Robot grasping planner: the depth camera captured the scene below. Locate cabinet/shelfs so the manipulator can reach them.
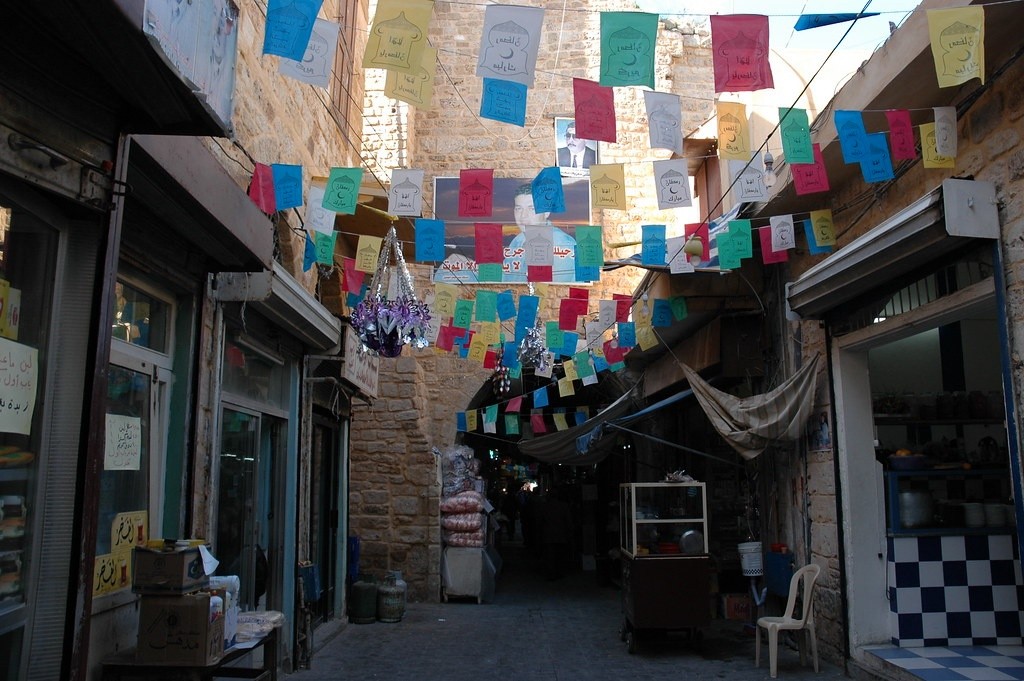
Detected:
[615,481,709,562]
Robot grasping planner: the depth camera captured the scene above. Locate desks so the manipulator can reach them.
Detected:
[98,627,277,681]
[443,544,490,604]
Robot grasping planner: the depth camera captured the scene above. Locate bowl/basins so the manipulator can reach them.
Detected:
[771,544,786,552]
[889,455,924,471]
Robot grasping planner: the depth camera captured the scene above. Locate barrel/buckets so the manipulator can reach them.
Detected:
[737,541,764,576]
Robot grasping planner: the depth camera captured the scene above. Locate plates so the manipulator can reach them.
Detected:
[679,530,703,553]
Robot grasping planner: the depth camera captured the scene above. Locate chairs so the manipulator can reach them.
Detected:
[755,564,821,678]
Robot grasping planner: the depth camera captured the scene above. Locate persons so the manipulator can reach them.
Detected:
[537,488,575,580]
[520,506,529,544]
[558,122,595,168]
[502,183,585,283]
[500,497,516,540]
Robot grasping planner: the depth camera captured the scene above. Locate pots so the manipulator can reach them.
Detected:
[899,491,932,527]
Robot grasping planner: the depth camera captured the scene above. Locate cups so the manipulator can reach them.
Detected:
[781,547,787,553]
[1007,506,1016,523]
[965,503,984,527]
[985,505,1005,527]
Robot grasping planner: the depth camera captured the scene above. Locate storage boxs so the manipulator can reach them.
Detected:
[134,575,237,666]
[130,541,211,596]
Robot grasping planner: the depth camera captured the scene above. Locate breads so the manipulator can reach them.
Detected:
[0,443,34,585]
